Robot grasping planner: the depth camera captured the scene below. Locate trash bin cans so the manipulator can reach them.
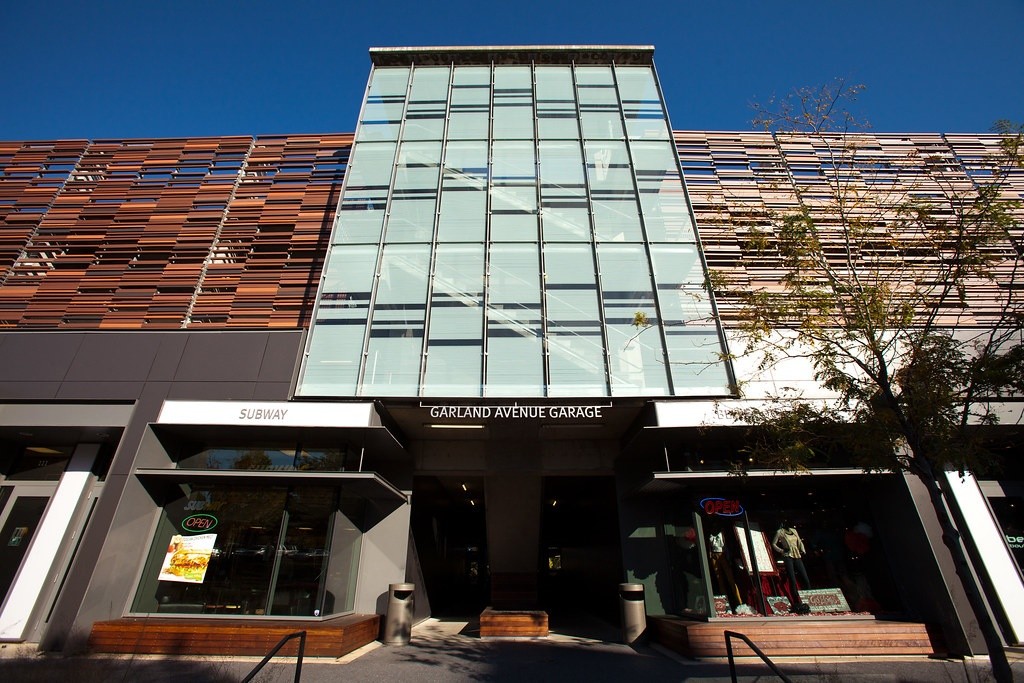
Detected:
[619,583,647,646]
[383,584,414,646]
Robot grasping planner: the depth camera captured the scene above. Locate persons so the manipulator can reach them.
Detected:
[709,521,742,606]
[772,517,812,613]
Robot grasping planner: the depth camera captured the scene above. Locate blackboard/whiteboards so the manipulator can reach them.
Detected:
[731,517,780,577]
